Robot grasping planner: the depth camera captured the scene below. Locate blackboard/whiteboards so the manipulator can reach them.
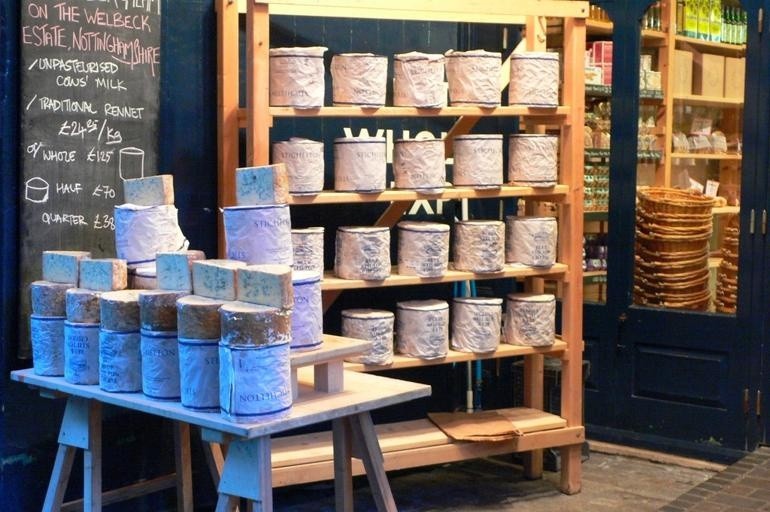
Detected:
[19,1,161,360]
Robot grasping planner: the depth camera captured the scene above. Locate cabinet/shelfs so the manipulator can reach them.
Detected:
[676,35,744,268]
[213,1,587,511]
[585,2,672,306]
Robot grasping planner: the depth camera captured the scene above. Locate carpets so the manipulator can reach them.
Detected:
[676,446,769,499]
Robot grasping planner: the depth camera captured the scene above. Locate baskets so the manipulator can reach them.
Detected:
[713,226,741,314]
[638,203,714,227]
[635,219,713,236]
[635,228,713,252]
[638,188,715,214]
[632,264,713,312]
[634,240,710,263]
[634,251,709,274]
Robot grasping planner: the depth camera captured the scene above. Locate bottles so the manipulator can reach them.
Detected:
[721,6,748,45]
[590,5,610,22]
[641,2,660,32]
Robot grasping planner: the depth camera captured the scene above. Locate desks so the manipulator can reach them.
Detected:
[286,334,371,400]
[11,367,433,511]
